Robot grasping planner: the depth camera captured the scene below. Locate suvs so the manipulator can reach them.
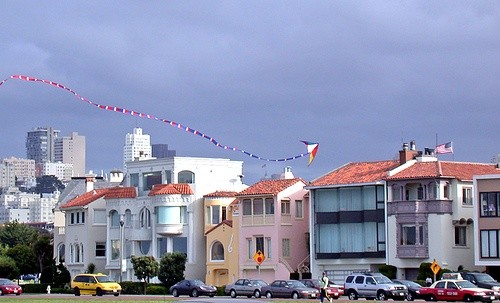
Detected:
[344,273,408,300]
[461,273,500,299]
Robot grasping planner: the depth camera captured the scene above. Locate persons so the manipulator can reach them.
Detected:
[43,285,58,298]
[320,271,332,303]
[426,274,432,286]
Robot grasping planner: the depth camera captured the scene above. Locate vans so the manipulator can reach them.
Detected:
[441,272,462,280]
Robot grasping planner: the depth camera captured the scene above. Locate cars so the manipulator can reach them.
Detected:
[20,274,36,281]
[70,274,122,296]
[169,279,216,297]
[0,278,22,295]
[391,279,423,301]
[297,278,342,299]
[327,281,345,295]
[262,280,320,299]
[420,280,491,302]
[225,279,268,298]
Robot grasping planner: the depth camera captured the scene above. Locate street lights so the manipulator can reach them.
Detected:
[120,221,124,272]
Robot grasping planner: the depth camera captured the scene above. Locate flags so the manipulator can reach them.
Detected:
[436,140,453,153]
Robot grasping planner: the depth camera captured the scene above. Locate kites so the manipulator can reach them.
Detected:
[298,139,319,166]
